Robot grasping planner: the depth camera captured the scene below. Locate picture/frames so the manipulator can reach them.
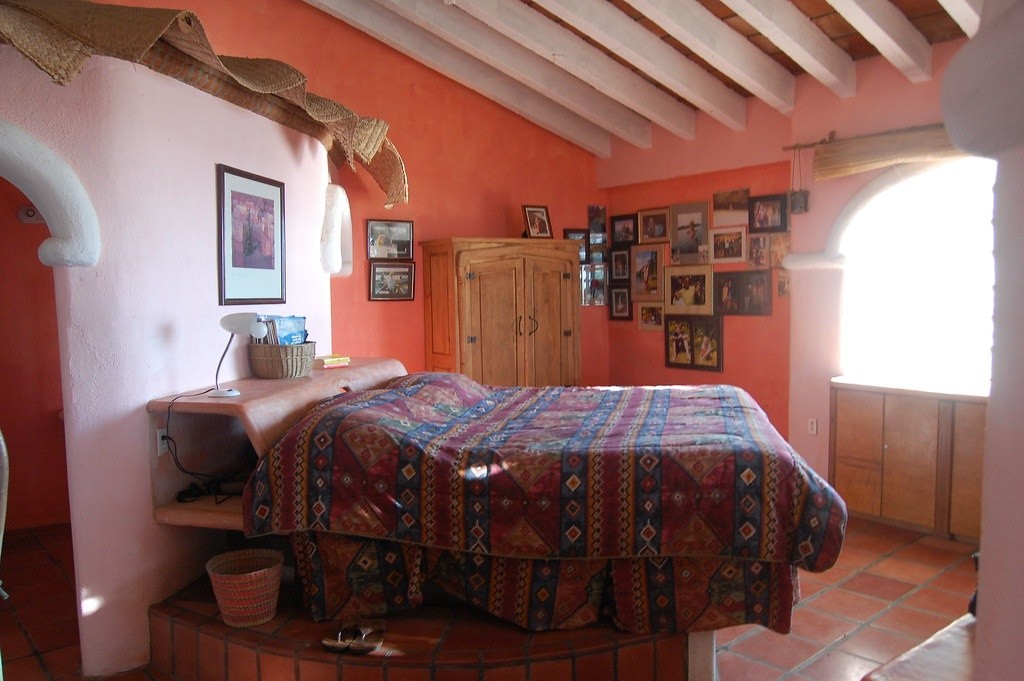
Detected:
[608,284,633,321]
[629,245,664,302]
[606,247,630,284]
[709,226,747,264]
[370,260,416,302]
[521,204,553,239]
[638,206,670,245]
[670,200,709,266]
[748,194,787,233]
[664,266,713,315]
[663,315,723,372]
[580,262,608,307]
[216,163,287,305]
[610,213,638,246]
[366,219,413,261]
[713,272,773,317]
[563,228,591,265]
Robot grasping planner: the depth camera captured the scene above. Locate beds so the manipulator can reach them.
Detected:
[146,356,847,636]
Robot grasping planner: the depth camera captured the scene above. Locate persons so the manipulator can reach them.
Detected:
[378,271,403,295]
[532,212,548,234]
[370,234,395,257]
[614,201,788,361]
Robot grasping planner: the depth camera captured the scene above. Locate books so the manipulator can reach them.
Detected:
[250,314,309,345]
[316,354,351,370]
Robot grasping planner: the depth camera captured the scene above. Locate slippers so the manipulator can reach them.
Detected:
[349,619,386,652]
[322,618,368,650]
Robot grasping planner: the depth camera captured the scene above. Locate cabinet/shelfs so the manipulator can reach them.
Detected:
[418,236,585,387]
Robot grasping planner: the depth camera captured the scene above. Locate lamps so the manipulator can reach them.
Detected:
[208,313,268,398]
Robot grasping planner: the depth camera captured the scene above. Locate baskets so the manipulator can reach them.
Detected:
[249,341,316,379]
[206,549,283,627]
